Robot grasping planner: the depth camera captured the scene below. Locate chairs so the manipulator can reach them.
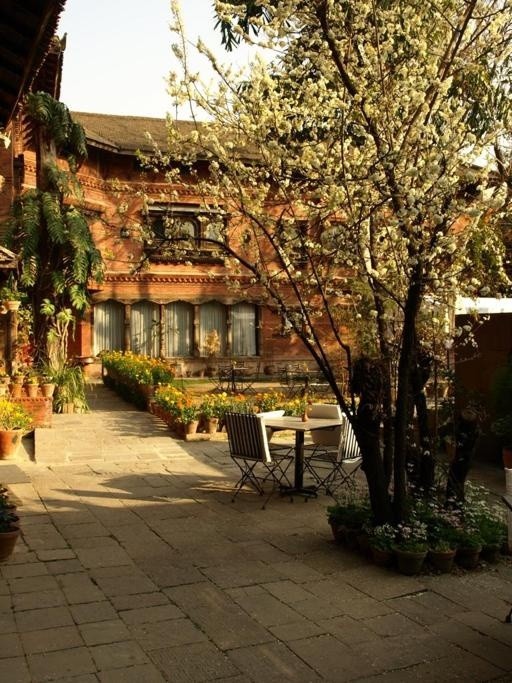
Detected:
[224,404,363,510]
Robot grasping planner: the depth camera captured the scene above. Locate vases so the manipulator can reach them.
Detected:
[376,547,482,573]
[151,404,219,439]
[328,521,352,544]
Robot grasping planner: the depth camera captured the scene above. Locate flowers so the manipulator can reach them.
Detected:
[327,477,506,549]
[147,386,303,419]
[100,349,176,384]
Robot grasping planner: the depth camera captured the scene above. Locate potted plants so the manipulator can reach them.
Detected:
[0,365,56,396]
[3,287,21,312]
[0,484,20,561]
[0,396,33,460]
